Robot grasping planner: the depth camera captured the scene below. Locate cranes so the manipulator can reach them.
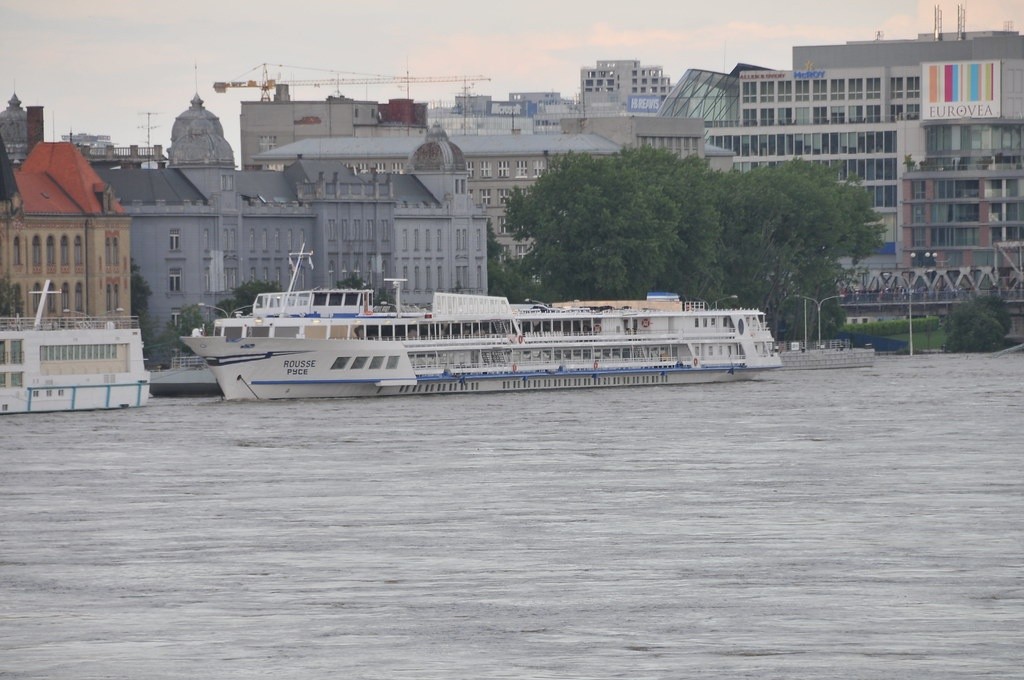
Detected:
[212,61,494,102]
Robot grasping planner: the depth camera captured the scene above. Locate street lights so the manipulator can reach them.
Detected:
[521,296,581,372]
[195,300,260,320]
[794,293,846,350]
[692,294,740,355]
[882,268,935,359]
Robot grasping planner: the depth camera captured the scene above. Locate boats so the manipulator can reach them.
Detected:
[180,237,784,404]
[1,279,152,417]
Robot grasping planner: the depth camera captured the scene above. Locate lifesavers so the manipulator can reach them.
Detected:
[594,325,602,333]
[642,318,650,328]
[593,362,598,369]
[518,334,523,344]
[512,364,517,372]
[694,358,697,365]
